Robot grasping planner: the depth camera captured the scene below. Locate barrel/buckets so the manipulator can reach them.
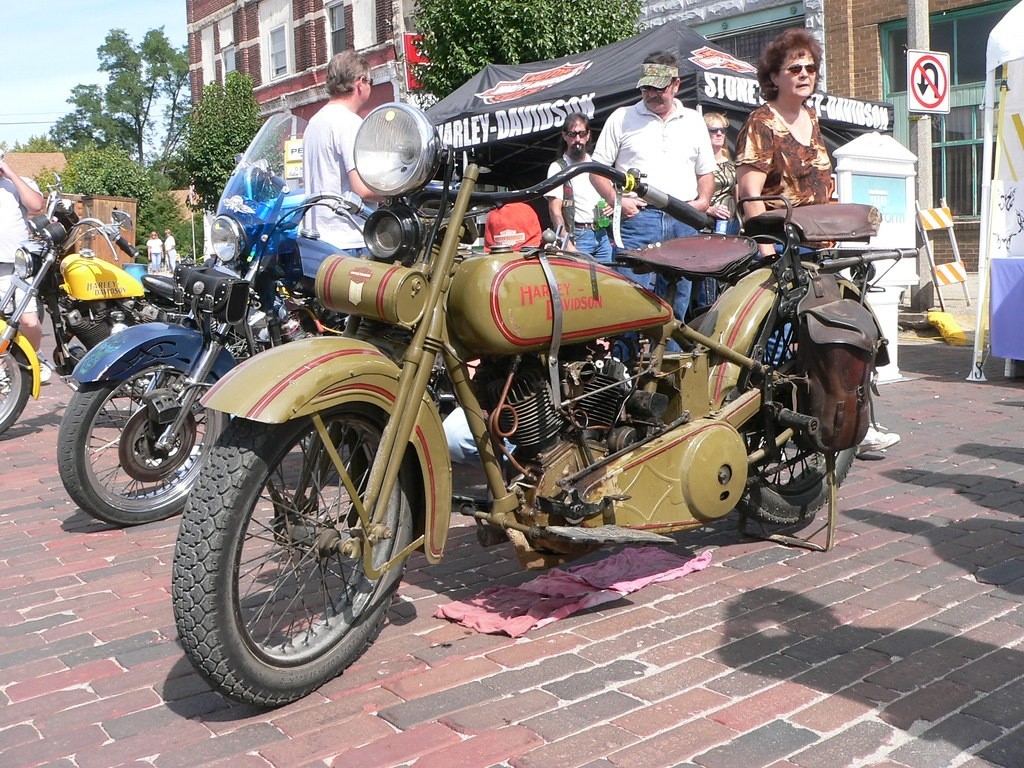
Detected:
[122,264,148,290]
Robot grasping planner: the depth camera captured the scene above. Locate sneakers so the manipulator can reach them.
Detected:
[853,423,900,457]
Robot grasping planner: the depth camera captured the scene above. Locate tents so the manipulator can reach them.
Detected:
[422,21,896,190]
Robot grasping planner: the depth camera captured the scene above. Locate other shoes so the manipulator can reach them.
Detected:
[0,369,8,386]
[37,351,54,383]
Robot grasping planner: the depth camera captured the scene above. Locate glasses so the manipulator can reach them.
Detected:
[358,77,374,88]
[708,127,730,134]
[562,130,588,139]
[641,86,668,93]
[779,64,818,75]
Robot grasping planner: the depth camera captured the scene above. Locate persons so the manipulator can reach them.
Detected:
[164,229,176,275]
[442,202,544,507]
[0,148,52,382]
[543,113,613,271]
[701,112,739,308]
[302,52,383,263]
[147,231,163,274]
[590,52,718,364]
[731,25,902,456]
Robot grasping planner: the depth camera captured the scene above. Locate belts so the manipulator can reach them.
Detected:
[637,204,652,209]
[575,223,596,230]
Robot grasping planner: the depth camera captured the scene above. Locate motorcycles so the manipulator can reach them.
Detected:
[1,101,922,710]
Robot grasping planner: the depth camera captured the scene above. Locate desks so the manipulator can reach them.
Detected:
[989,256,1024,378]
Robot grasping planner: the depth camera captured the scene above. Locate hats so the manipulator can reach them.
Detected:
[636,64,679,91]
[484,202,541,253]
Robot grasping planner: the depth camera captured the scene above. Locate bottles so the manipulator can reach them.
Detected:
[598,198,610,227]
[715,207,728,234]
